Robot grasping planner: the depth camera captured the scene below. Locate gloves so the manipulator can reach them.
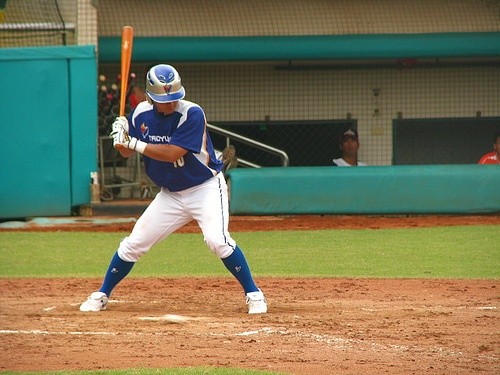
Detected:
[109,126,138,150]
[112,116,129,134]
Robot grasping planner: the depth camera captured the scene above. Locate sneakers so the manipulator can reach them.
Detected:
[245,288,267,314]
[79,291,109,312]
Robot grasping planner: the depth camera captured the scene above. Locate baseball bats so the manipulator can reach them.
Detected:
[121,26,135,121]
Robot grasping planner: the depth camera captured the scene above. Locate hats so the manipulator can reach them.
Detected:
[340,128,358,143]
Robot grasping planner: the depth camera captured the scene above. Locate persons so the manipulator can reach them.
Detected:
[80,63,267,315]
[476,132,500,164]
[325,128,368,167]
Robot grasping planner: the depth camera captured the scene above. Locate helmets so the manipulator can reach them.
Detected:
[145,63,186,105]
